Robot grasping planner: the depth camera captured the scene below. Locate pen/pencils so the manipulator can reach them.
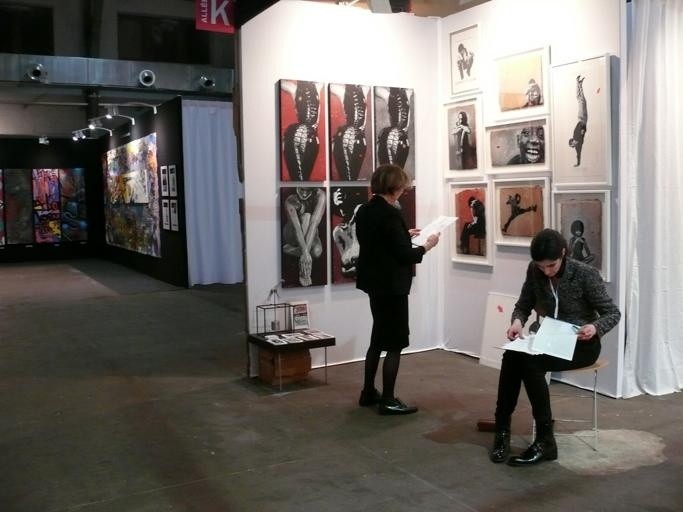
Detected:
[573,326,579,330]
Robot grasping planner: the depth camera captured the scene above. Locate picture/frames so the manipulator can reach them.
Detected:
[547,52,614,187]
[492,175,551,249]
[478,292,552,386]
[483,115,553,175]
[551,190,614,283]
[451,179,495,267]
[483,44,552,130]
[443,23,488,104]
[443,93,485,179]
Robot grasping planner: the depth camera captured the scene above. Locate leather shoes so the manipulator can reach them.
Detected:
[359,389,381,405]
[379,397,418,415]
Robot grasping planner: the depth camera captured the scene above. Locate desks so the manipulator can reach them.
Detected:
[249,330,337,392]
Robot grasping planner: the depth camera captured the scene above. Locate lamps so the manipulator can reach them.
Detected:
[69,102,162,143]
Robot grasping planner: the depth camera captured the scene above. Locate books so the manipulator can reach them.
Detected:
[410,215,458,247]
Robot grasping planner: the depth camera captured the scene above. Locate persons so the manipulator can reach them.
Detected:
[488,229,620,468]
[281,188,326,288]
[452,111,476,169]
[350,164,439,416]
[566,218,594,266]
[524,78,544,106]
[332,189,364,276]
[456,44,474,81]
[456,196,486,255]
[569,74,587,167]
[506,126,545,164]
[503,193,535,230]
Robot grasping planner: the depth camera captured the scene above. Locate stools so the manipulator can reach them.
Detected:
[529,355,611,450]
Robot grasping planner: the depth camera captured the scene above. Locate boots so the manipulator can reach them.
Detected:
[507,420,557,466]
[490,420,510,462]
